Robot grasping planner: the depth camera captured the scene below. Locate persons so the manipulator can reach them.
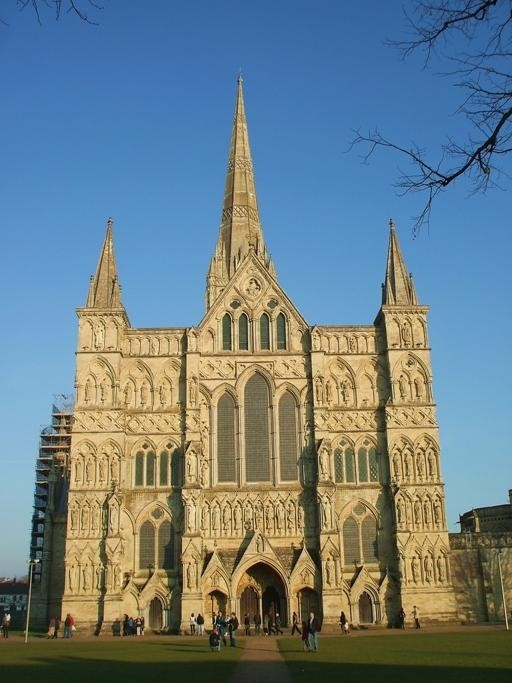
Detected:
[412,605,421,629]
[123,615,145,636]
[1,612,11,639]
[48,613,74,639]
[340,611,345,634]
[344,620,350,634]
[189,611,318,652]
[399,608,405,630]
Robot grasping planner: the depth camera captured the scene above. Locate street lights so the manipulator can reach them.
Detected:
[495,550,509,630]
[21,558,41,643]
